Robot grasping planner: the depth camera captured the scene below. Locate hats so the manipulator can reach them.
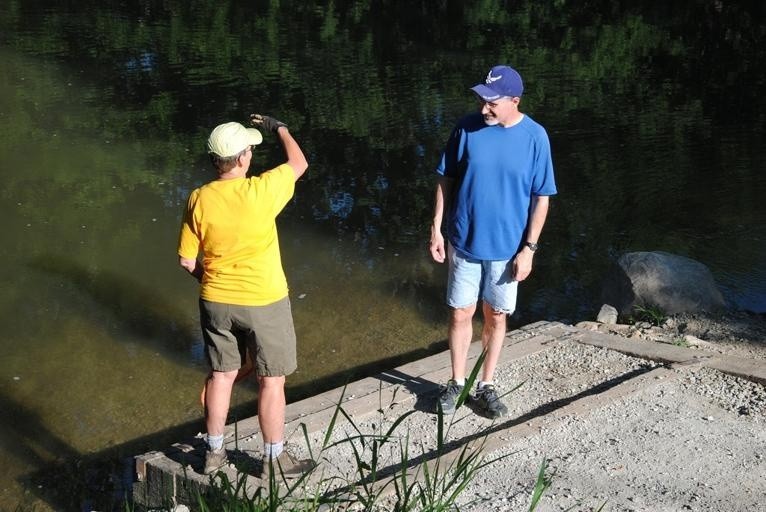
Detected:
[468,64,525,104]
[206,120,264,158]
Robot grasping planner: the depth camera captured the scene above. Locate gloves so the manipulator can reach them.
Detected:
[248,113,288,133]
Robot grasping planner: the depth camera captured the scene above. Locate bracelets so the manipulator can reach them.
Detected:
[524,241,538,251]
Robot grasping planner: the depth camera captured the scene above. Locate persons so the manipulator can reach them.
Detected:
[177,113,317,481]
[426,64,559,419]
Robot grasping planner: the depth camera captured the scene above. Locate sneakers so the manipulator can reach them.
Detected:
[474,380,510,420]
[259,447,318,483]
[429,376,470,415]
[203,441,256,476]
